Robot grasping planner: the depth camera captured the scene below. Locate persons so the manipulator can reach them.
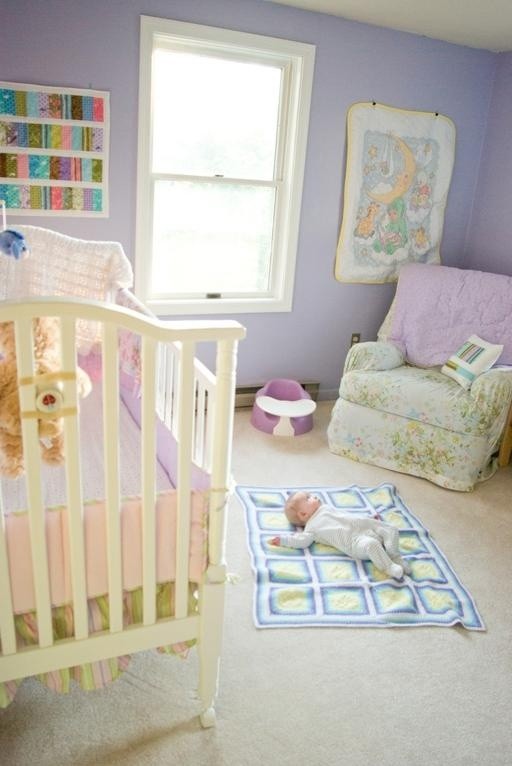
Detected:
[269,490,412,579]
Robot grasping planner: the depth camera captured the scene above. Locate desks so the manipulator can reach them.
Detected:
[254,393,318,438]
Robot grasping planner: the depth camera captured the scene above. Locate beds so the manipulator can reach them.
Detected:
[0,221,248,729]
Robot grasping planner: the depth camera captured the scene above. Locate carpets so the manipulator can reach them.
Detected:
[233,475,490,636]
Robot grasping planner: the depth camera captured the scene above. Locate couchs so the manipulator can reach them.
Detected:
[322,260,512,492]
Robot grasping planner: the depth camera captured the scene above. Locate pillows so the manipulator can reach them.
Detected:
[442,331,505,389]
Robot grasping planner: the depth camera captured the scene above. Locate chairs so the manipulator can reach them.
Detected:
[249,377,318,438]
